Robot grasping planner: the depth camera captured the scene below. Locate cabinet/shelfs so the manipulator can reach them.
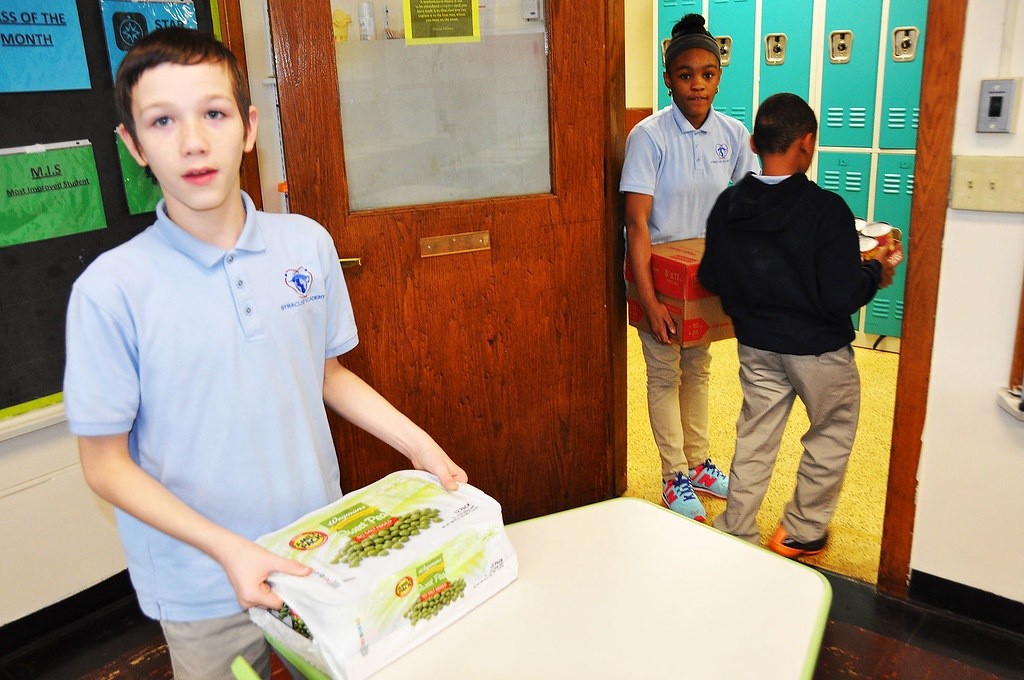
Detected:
[653,1,927,353]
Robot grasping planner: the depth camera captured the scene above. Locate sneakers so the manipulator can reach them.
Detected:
[660,474,708,524]
[768,520,829,560]
[684,458,731,502]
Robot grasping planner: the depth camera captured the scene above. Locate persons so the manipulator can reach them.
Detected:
[62,25,468,680]
[698,92,896,558]
[618,10,764,524]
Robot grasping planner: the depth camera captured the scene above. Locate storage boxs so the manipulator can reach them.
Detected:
[628,237,735,349]
[246,465,519,680]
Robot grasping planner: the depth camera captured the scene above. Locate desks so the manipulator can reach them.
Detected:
[230,496,834,680]
